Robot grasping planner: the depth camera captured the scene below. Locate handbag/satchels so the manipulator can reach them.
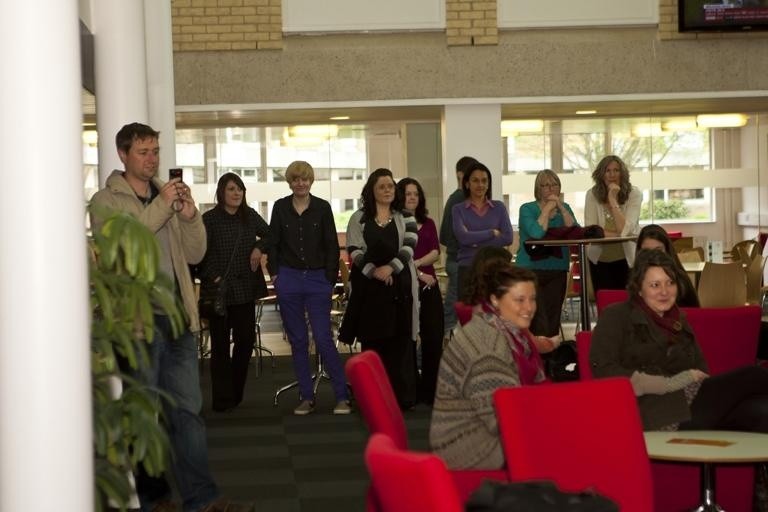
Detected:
[200,279,225,317]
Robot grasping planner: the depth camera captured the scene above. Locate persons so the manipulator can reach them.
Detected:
[266,161,352,414]
[590,249,768,511]
[635,224,702,308]
[89,121,254,512]
[585,156,643,292]
[340,168,444,410]
[514,169,578,375]
[439,157,513,335]
[196,173,274,413]
[461,477,622,511]
[429,246,563,471]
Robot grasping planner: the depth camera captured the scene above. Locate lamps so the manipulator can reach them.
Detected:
[630,114,750,139]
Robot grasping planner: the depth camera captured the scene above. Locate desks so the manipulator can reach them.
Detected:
[644,430,768,511]
[682,261,747,273]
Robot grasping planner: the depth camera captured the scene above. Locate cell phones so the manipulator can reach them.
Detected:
[169,168,182,182]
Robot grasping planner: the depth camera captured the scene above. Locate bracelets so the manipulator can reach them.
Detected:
[612,203,620,208]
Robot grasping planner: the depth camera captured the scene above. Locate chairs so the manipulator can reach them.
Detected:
[672,236,694,252]
[675,247,704,262]
[747,254,762,305]
[193,223,450,407]
[491,377,654,512]
[730,240,762,263]
[365,431,461,512]
[344,350,408,448]
[681,304,761,374]
[696,261,748,306]
[576,330,592,379]
[596,288,630,313]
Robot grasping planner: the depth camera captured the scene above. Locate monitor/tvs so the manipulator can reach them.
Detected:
[678,0,768,33]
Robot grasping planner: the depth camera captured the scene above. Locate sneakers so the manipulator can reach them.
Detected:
[333,400,352,414]
[294,400,316,414]
